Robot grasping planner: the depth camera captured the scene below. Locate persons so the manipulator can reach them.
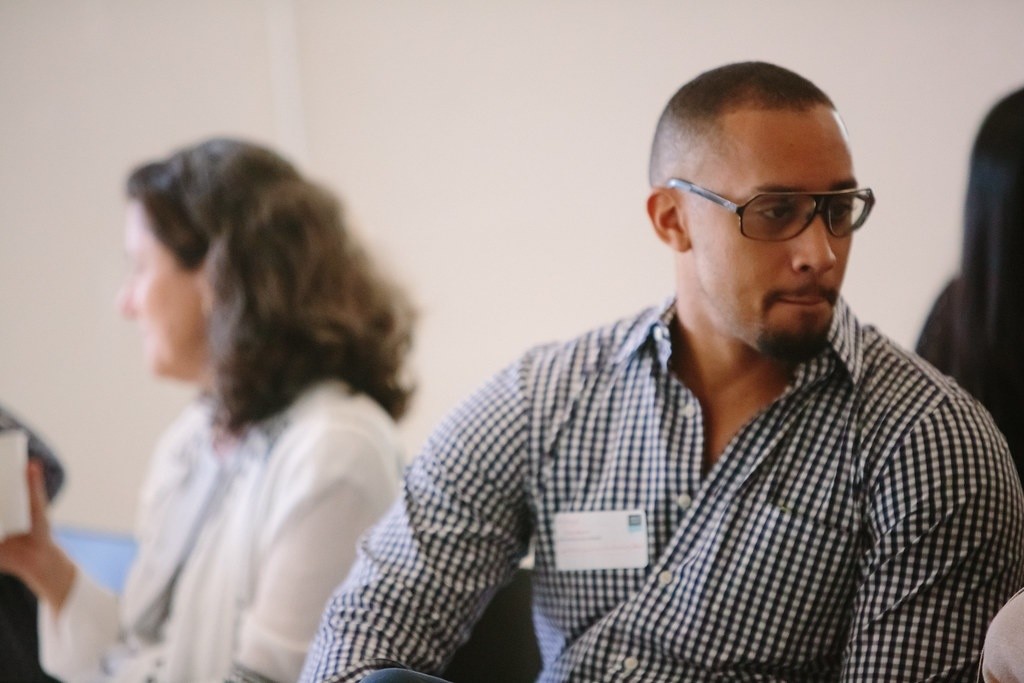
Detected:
[302,61,1022,683]
[0,140,411,683]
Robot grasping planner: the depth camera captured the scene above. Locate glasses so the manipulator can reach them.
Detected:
[666,179,875,243]
